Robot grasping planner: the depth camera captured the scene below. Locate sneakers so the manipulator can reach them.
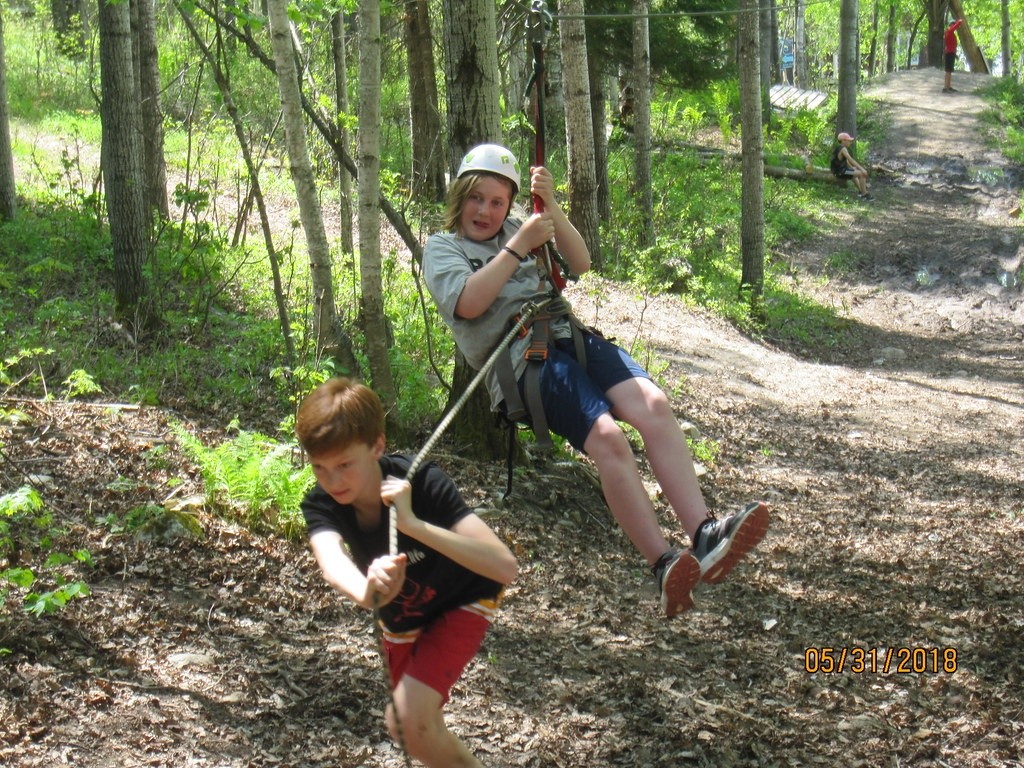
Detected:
[651,551,701,619]
[696,503,770,584]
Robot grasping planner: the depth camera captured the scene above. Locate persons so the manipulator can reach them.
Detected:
[942,19,963,93]
[831,132,874,200]
[619,79,633,115]
[422,144,770,616]
[295,378,518,768]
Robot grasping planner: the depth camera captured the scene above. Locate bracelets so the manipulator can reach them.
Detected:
[502,246,524,262]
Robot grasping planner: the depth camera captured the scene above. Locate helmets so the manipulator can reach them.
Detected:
[456,144,522,191]
